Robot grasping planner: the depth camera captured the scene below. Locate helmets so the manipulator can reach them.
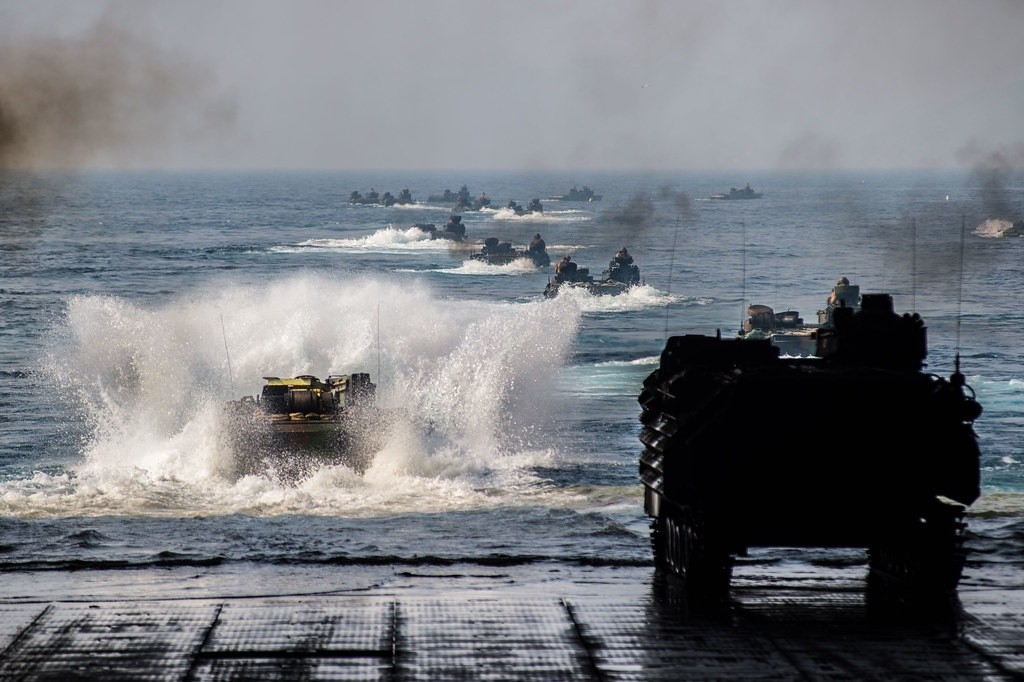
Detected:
[838,277,850,285]
[564,255,571,260]
[619,247,627,253]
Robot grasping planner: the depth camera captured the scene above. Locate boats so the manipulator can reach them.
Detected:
[347,181,604,217]
[543,258,647,298]
[219,368,409,480]
[735,302,824,357]
[409,221,468,243]
[469,237,551,269]
[712,188,764,202]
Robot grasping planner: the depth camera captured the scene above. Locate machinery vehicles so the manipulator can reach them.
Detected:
[646,219,985,642]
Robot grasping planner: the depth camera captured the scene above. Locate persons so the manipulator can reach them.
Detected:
[532,233,545,244]
[616,247,630,259]
[481,193,487,200]
[554,256,571,272]
[830,277,861,304]
